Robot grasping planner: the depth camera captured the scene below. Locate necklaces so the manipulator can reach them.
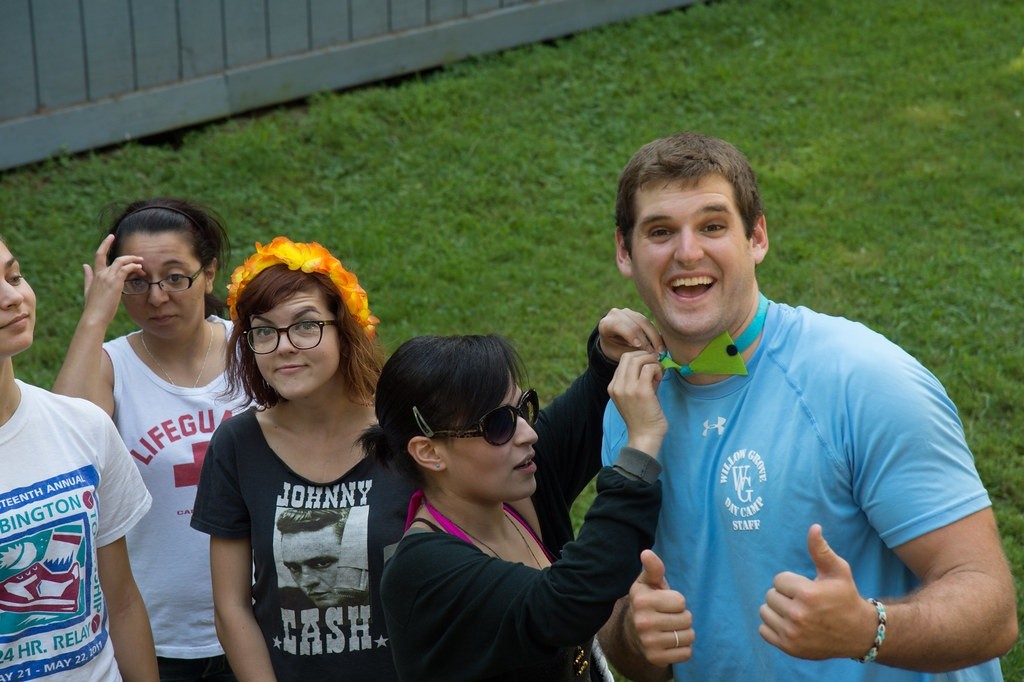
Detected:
[142,321,213,388]
[434,510,542,570]
[659,292,767,375]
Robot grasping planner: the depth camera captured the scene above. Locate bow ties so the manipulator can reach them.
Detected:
[656,293,768,376]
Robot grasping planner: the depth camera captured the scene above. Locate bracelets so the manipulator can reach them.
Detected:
[851,598,886,665]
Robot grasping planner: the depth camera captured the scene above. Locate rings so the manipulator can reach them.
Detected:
[674,631,679,647]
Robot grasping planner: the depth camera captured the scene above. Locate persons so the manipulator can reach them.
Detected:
[191,237,407,681]
[0,241,163,682]
[52,200,265,682]
[354,308,667,682]
[590,136,1019,682]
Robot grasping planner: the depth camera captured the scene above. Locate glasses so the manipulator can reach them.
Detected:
[119,265,207,295]
[426,390,540,446]
[243,320,338,355]
